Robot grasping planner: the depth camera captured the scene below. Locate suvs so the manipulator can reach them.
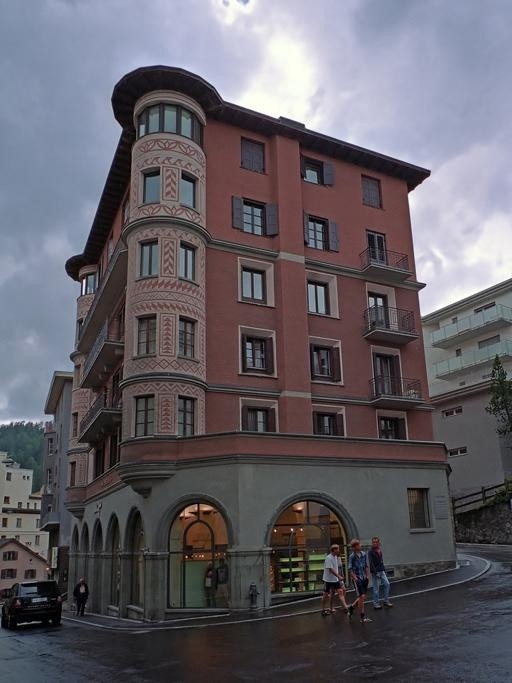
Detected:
[1,579,63,631]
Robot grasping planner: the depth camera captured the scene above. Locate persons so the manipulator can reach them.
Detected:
[70,575,90,615]
[321,544,350,617]
[215,556,229,604]
[345,537,374,624]
[202,560,218,607]
[329,551,345,613]
[369,537,393,609]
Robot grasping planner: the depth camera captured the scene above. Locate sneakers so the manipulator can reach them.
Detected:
[373,600,393,609]
[344,605,375,625]
[321,607,336,615]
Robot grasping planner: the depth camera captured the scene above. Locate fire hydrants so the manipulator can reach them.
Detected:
[249,584,261,609]
